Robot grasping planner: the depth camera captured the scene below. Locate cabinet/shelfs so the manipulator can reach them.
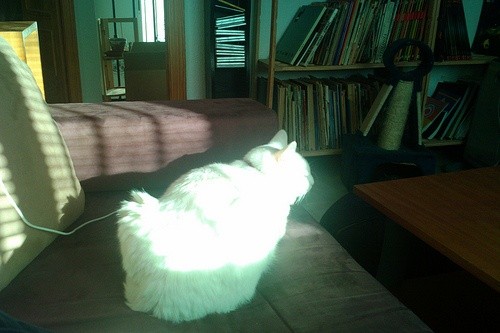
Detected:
[251,0,500,157]
[98,18,139,101]
[0,22,46,100]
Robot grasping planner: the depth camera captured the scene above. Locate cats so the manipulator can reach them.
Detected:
[115,129,315,324]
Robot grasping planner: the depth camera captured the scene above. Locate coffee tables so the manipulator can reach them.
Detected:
[354,167,500,293]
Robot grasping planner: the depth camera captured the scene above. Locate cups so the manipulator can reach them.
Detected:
[110,38,126,52]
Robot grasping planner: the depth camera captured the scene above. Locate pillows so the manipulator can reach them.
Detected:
[1,36,87,292]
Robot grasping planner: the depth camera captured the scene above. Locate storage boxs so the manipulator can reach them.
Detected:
[124,41,170,100]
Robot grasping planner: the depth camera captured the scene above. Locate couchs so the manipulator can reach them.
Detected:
[0,36,433,333]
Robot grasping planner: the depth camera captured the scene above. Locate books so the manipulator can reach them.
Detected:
[214,0,246,68]
[272,0,480,154]
